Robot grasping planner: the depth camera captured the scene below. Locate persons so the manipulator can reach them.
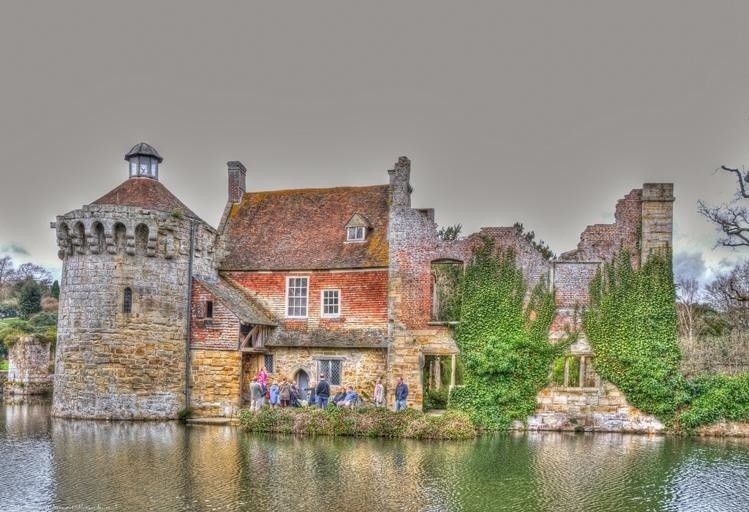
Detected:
[395,376,408,411]
[249,366,298,413]
[316,374,330,409]
[373,378,387,408]
[331,387,347,407]
[336,386,356,410]
